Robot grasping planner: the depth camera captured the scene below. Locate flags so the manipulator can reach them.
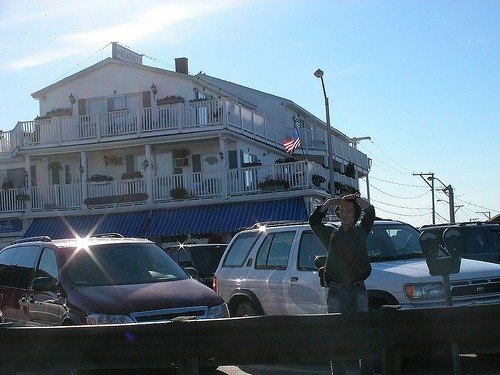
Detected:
[280,128,302,156]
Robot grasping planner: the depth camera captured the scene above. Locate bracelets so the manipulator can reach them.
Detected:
[355,194,358,201]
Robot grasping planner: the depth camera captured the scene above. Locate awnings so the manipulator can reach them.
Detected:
[21,196,310,242]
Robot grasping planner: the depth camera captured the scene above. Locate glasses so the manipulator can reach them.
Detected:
[338,210,355,214]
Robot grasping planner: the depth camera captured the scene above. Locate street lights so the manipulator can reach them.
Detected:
[314,69,335,196]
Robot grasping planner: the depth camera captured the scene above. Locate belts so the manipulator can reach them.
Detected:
[329,280,364,289]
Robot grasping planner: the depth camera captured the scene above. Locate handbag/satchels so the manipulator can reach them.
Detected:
[318,266,326,287]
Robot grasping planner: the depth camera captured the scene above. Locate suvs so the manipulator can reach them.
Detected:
[166,221,500,318]
[1,233,229,325]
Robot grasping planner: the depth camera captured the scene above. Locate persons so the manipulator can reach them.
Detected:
[309,193,376,375]
[2,177,14,210]
